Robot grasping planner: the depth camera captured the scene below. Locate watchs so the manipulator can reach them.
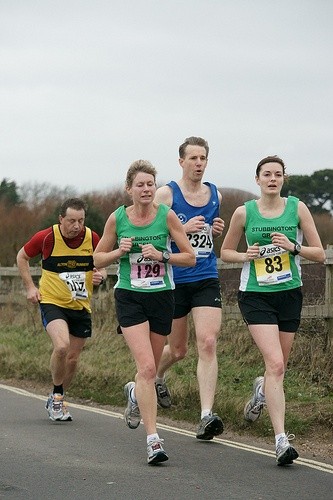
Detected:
[101,276,104,285]
[290,243,302,255]
[160,250,171,264]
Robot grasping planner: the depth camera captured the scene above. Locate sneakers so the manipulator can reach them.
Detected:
[244,375,267,423]
[196,411,224,440]
[277,431,299,464]
[155,378,172,409]
[145,439,168,464]
[59,402,72,421]
[45,393,64,421]
[124,382,142,429]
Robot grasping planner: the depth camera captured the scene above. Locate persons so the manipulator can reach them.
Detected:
[17,198,107,422]
[93,159,196,465]
[221,155,327,466]
[155,138,223,441]
